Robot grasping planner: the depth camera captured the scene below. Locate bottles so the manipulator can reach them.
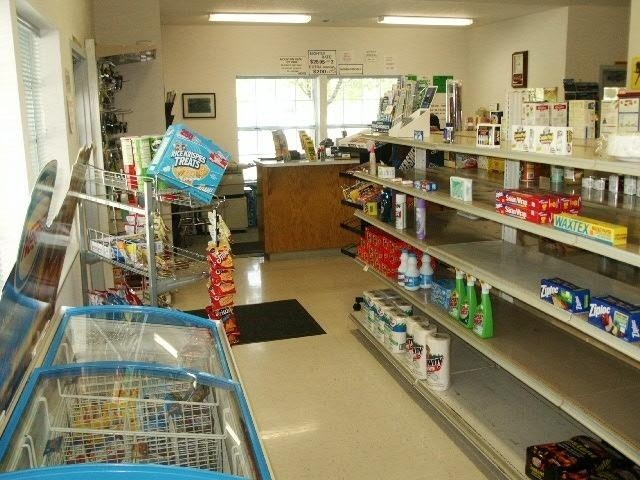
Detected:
[395,193,407,229]
[416,199,426,240]
[444,123,454,144]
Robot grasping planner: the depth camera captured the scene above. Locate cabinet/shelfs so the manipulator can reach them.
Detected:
[349,130,640,480]
[76,163,232,307]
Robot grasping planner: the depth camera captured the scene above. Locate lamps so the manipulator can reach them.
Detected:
[208,13,311,24]
[377,15,474,26]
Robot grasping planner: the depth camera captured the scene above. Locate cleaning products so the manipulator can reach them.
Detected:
[459,273,477,329]
[472,280,493,338]
[448,268,466,320]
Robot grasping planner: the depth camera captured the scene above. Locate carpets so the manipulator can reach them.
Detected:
[229,241,265,256]
[181,299,328,346]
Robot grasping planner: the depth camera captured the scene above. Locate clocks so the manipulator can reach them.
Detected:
[511,51,528,88]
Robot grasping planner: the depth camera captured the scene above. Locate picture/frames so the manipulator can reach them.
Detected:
[182,92,216,118]
